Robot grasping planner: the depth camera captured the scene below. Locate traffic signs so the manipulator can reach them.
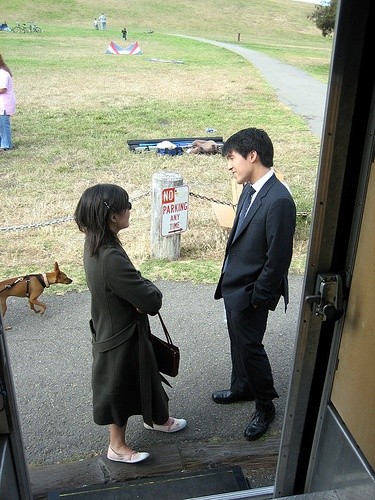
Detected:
[162,186,189,237]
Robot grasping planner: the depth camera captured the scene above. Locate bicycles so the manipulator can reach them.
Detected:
[11,21,42,33]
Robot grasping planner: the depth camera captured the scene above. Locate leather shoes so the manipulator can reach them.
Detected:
[244,405,275,441]
[212,388,255,403]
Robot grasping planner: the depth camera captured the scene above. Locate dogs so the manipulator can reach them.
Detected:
[0,262,73,330]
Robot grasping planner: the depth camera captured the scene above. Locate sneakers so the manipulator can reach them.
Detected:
[144,418,186,433]
[106,445,150,464]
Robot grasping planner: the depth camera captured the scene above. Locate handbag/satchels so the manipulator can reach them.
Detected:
[149,311,178,377]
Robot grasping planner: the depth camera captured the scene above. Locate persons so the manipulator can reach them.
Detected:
[0,54,17,150]
[122,28,127,40]
[212,127,296,441]
[73,184,187,462]
[94,18,99,30]
[98,12,106,30]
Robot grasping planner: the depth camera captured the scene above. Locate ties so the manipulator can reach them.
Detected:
[223,186,256,273]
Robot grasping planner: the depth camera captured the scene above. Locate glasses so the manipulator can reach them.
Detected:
[110,202,131,212]
